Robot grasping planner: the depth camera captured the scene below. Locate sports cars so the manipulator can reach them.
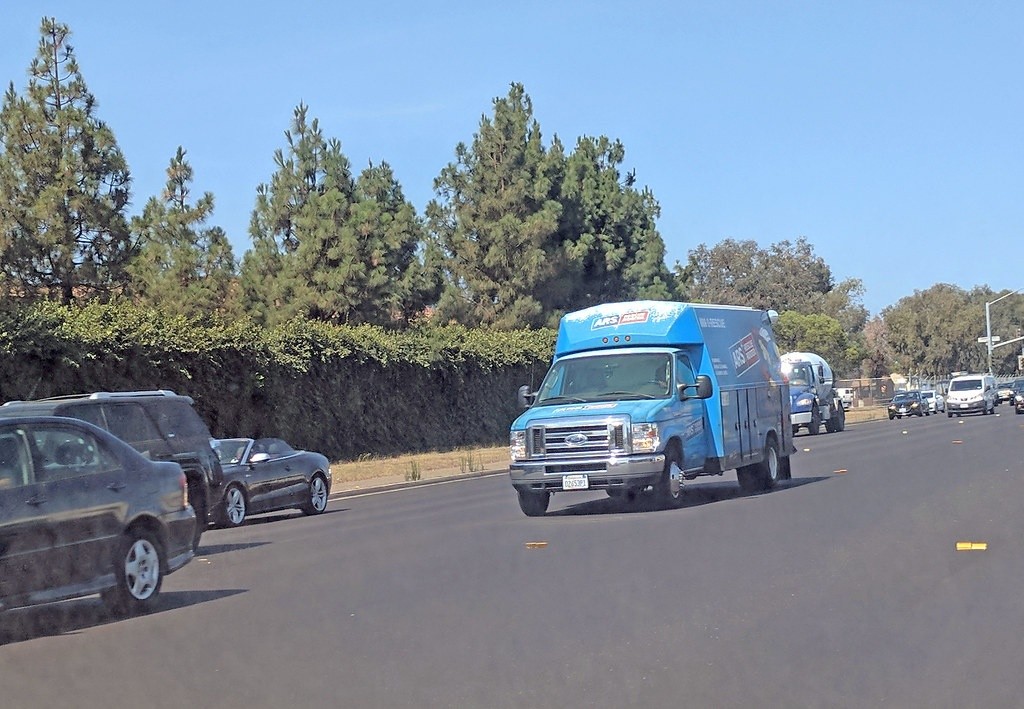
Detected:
[212,438,334,527]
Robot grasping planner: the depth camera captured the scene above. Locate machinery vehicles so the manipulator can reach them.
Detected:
[778,352,845,436]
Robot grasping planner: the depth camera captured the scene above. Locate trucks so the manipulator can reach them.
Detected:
[506,301,798,511]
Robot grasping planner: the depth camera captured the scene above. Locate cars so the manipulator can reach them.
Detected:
[1,414,199,622]
[920,390,946,414]
[995,377,1024,414]
[886,392,930,422]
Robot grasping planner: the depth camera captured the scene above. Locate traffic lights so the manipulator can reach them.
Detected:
[1018,355,1024,371]
[1016,327,1021,339]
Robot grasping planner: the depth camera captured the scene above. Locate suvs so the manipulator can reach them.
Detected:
[0,388,224,532]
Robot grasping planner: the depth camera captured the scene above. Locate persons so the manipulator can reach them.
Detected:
[238,445,259,459]
[913,394,917,398]
[654,367,681,392]
[0,465,17,488]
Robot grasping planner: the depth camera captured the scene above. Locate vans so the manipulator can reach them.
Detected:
[834,387,855,402]
[944,376,998,418]
